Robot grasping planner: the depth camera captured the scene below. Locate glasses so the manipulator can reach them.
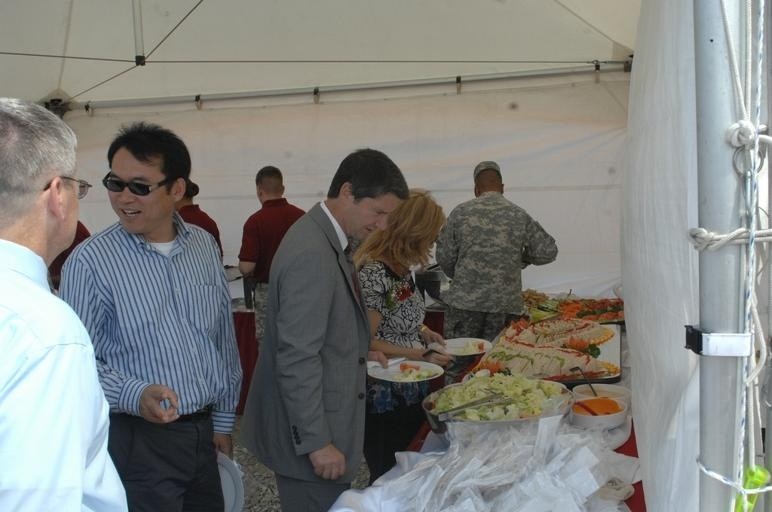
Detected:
[103,171,170,195]
[43,176,92,198]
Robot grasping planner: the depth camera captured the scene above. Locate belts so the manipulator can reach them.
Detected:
[173,403,213,423]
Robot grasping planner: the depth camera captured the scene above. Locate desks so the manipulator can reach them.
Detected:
[233,313,493,416]
[403,318,645,512]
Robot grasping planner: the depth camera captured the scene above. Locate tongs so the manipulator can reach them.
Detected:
[437,392,512,422]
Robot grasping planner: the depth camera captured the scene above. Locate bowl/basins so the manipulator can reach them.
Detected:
[421,379,576,451]
[568,398,627,431]
[572,384,631,409]
[423,264,453,306]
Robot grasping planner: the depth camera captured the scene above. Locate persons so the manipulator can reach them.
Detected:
[0,94,132,512]
[434,159,559,390]
[238,147,411,511]
[59,122,245,512]
[48,220,91,290]
[176,178,224,265]
[239,166,306,346]
[352,187,446,481]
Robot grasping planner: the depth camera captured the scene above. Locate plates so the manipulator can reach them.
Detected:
[217,452,244,512]
[427,337,493,356]
[367,361,445,383]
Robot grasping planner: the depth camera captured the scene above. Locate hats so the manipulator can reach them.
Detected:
[473,161,500,179]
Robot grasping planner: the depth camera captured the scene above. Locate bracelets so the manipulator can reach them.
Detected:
[419,324,428,333]
